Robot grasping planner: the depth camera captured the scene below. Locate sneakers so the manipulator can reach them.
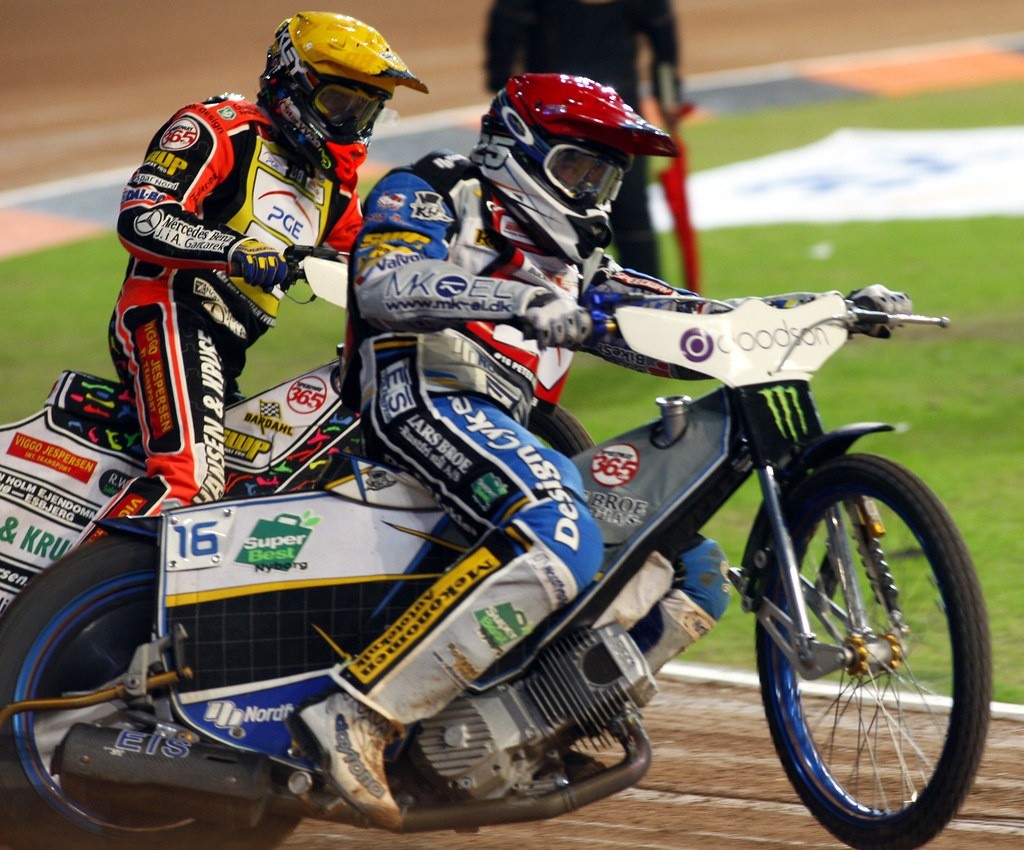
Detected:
[286,690,404,832]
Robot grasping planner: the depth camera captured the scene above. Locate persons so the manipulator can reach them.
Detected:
[481,0,681,284]
[87,10,428,541]
[283,71,912,832]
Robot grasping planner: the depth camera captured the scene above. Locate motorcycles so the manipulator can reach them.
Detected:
[0,290,993,850]
[1,237,597,612]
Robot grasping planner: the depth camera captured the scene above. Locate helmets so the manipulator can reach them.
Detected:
[258,9,429,185]
[470,74,679,265]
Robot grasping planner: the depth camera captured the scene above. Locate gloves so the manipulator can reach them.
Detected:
[523,290,593,351]
[232,239,290,294]
[847,284,912,339]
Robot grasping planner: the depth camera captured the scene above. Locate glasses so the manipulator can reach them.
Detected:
[277,29,382,136]
[493,90,625,210]
